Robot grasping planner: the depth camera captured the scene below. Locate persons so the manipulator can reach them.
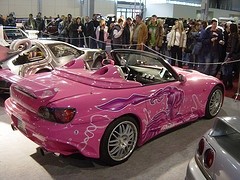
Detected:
[0,11,240,90]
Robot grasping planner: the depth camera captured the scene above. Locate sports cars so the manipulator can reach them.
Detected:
[0,49,225,165]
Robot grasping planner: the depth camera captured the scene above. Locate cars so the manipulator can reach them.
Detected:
[0,25,102,95]
